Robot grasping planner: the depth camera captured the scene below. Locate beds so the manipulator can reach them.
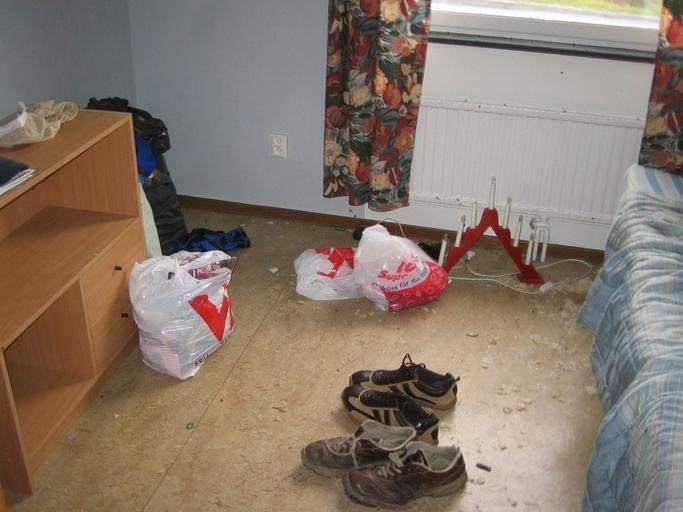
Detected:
[573,160,682,512]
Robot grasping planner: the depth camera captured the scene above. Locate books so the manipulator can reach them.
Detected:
[0,157,36,197]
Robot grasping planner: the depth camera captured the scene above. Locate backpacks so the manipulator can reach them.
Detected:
[84,97,190,256]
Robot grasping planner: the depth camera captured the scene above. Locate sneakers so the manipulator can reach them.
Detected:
[349,352,460,410]
[301,419,419,478]
[343,441,467,509]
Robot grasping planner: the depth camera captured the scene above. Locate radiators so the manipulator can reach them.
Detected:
[407,95,646,227]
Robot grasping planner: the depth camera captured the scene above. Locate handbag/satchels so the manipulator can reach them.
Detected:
[341,385,438,446]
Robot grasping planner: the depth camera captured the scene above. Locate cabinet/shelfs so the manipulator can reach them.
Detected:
[0,102,153,512]
